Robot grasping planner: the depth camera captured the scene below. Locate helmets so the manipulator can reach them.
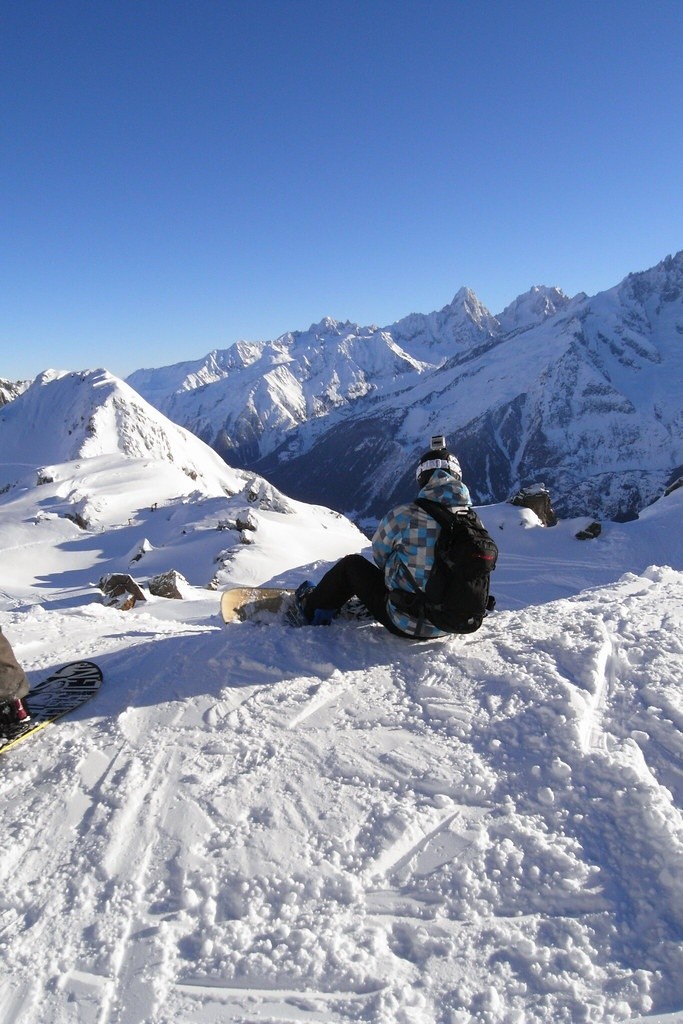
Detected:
[416,450,462,488]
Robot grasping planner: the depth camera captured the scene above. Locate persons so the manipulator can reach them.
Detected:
[285,451,498,638]
[0,626,32,724]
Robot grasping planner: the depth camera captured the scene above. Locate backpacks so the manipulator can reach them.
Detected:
[390,498,499,634]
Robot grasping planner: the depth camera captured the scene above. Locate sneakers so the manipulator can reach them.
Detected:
[0,698,32,725]
[295,580,337,626]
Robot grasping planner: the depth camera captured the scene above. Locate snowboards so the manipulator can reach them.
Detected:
[221,590,353,625]
[0,663,104,753]
